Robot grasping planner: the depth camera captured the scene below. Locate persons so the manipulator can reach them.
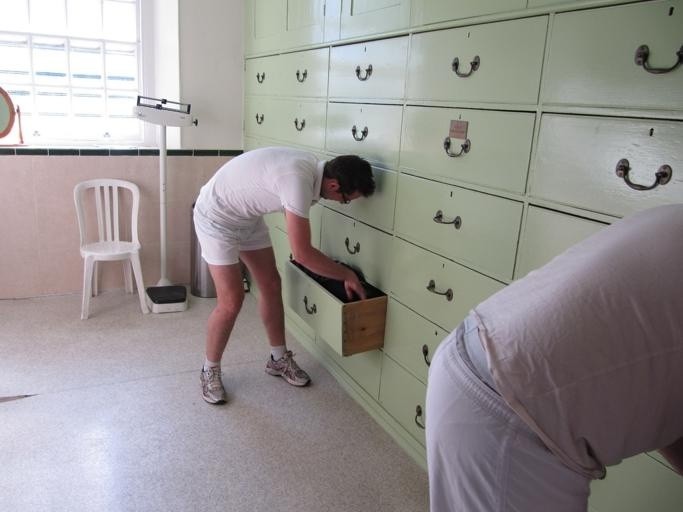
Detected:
[192,146,377,406]
[422,201,683,512]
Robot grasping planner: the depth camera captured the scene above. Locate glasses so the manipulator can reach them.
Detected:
[341,193,351,204]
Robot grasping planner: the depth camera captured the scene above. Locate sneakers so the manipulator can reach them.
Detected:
[265,350,311,386]
[200,365,227,404]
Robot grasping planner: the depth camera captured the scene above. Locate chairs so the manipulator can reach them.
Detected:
[71,177,150,320]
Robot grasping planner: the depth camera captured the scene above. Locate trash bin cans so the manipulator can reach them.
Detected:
[187,202,216,299]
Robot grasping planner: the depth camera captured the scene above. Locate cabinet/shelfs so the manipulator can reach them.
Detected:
[241,0,683,512]
